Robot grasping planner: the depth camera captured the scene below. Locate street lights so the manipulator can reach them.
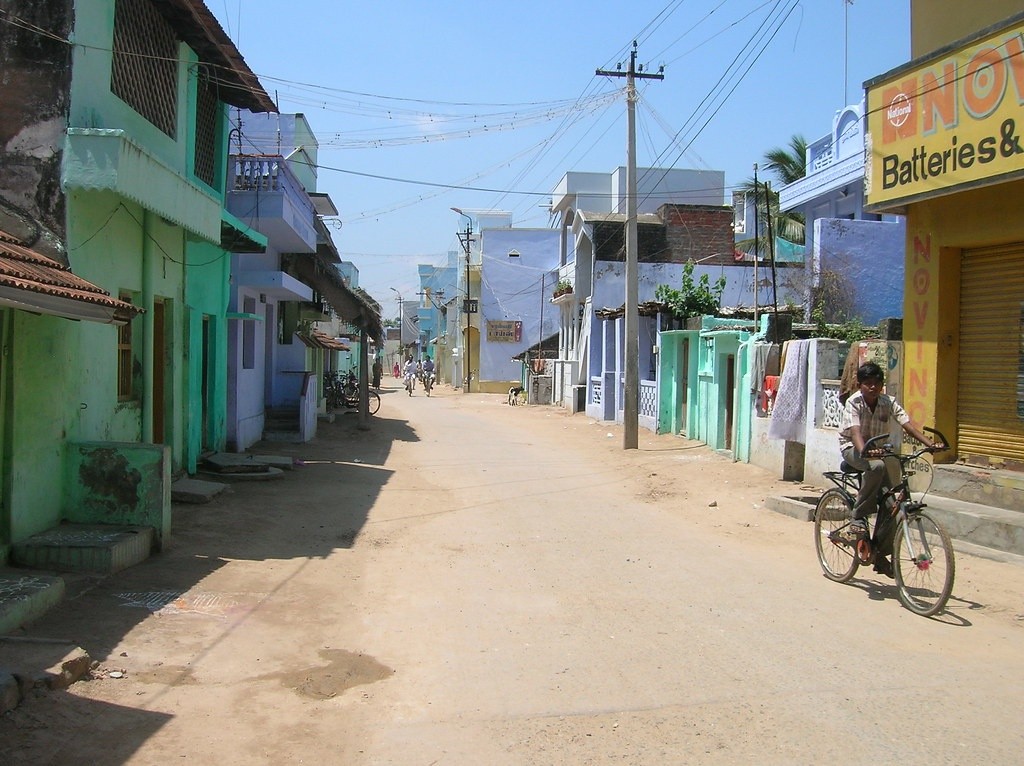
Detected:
[391,287,402,378]
[451,207,475,392]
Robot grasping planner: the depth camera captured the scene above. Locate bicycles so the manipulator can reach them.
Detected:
[402,372,418,397]
[422,371,436,396]
[813,426,955,617]
[416,369,423,384]
[324,370,380,414]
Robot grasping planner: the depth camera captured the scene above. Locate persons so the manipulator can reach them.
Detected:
[837,360,944,580]
[393,361,399,379]
[403,354,418,390]
[422,355,436,390]
[372,358,383,390]
[416,359,424,384]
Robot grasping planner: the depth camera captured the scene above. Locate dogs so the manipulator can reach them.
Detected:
[508,385,525,406]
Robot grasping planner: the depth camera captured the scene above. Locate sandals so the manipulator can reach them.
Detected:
[874,557,894,579]
[851,517,866,533]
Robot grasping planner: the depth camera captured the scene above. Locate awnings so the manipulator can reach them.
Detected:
[219,209,268,254]
[60,127,222,245]
[281,253,384,430]
[152,0,282,116]
